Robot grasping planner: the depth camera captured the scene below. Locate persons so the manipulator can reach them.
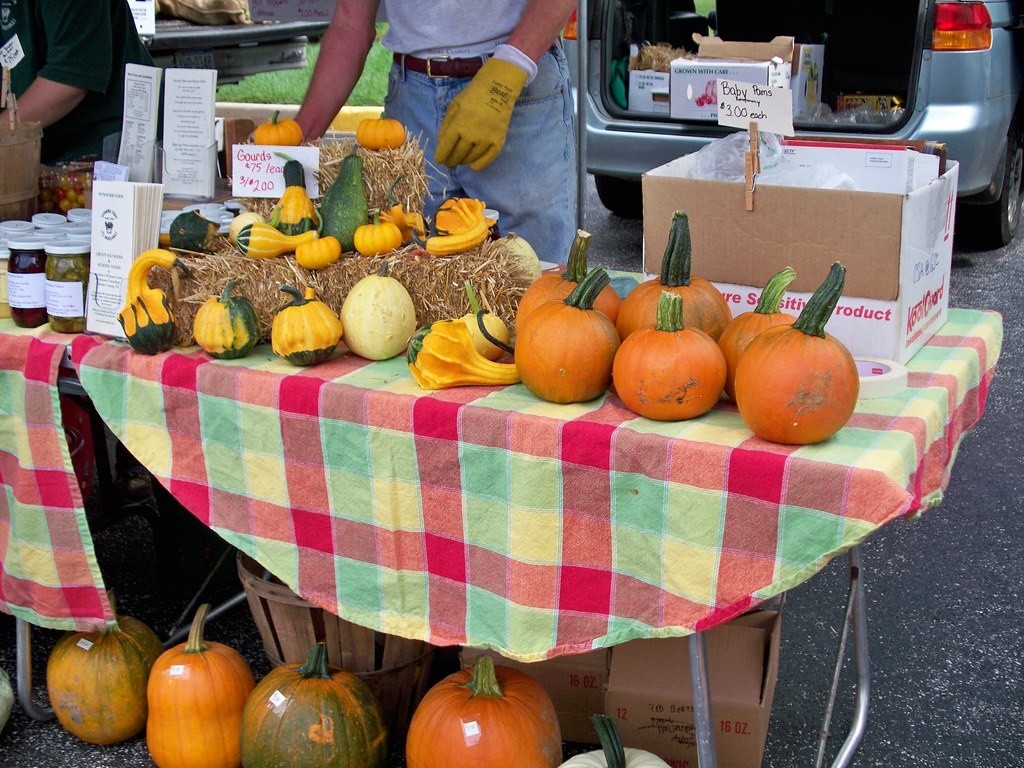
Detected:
[287,0,580,276]
[0,0,163,510]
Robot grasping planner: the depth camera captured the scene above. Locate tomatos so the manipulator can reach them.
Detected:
[37,171,90,212]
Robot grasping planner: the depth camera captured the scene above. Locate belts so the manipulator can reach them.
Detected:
[393,50,493,79]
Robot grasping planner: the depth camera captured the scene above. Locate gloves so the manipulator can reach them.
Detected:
[433,58,527,173]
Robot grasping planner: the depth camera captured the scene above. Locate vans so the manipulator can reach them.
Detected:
[585,0,1024,250]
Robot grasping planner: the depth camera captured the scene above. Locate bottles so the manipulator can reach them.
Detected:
[484,209,501,242]
[0,209,92,333]
[159,198,246,246]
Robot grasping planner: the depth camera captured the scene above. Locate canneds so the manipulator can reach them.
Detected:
[0,207,93,334]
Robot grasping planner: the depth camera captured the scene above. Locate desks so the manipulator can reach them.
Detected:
[0,262,1002,768]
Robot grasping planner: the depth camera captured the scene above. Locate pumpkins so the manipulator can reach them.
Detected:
[405,653,563,768]
[46,586,167,744]
[557,714,673,768]
[119,107,543,392]
[241,642,390,768]
[0,667,15,737]
[146,604,254,768]
[515,209,860,446]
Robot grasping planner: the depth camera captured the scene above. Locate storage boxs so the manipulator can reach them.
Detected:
[628,43,671,115]
[669,33,795,125]
[788,44,824,121]
[641,138,959,368]
[605,605,783,768]
[836,94,904,120]
[460,641,611,744]
